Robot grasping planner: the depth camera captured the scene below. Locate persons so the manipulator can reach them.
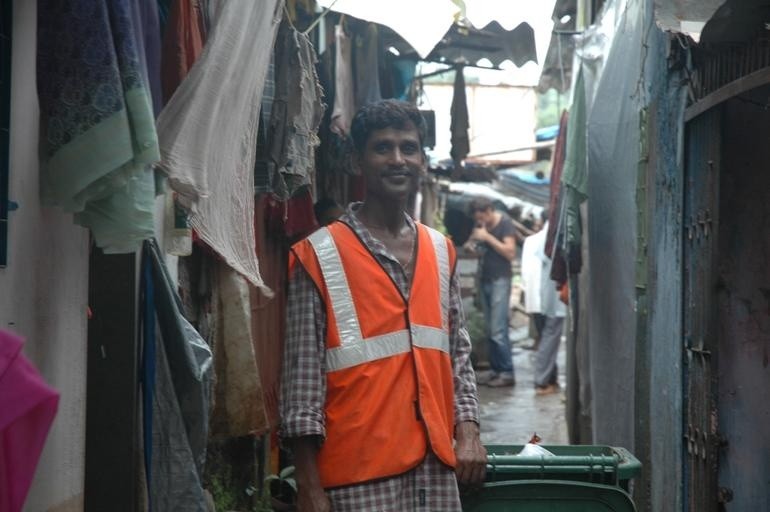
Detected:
[519,314,541,351]
[462,196,517,387]
[315,197,345,227]
[520,209,567,396]
[275,97,488,512]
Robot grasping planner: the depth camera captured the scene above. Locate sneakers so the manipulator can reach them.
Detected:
[480,371,515,386]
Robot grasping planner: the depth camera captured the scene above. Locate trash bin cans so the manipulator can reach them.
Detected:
[458,442,643,511]
